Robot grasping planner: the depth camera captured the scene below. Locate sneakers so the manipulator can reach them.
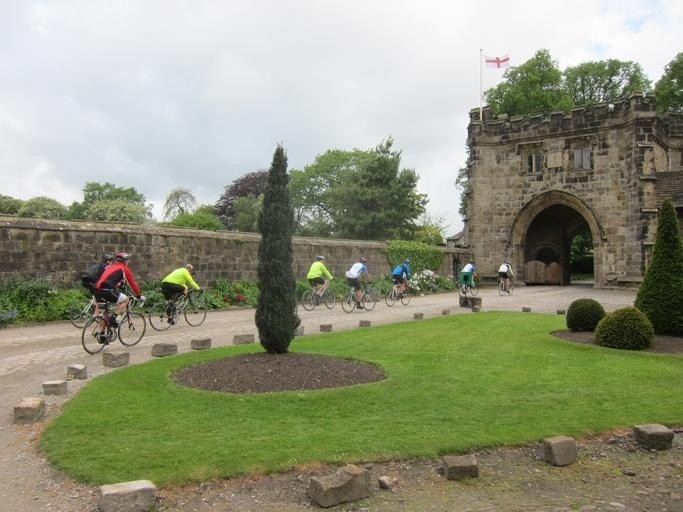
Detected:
[168,318,174,325]
[110,317,118,328]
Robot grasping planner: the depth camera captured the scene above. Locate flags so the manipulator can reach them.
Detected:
[482,52,511,69]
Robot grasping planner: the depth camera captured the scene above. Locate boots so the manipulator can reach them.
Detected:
[357,302,363,309]
[400,293,403,298]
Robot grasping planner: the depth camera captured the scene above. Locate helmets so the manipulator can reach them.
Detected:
[404,260,410,265]
[102,253,130,260]
[360,257,367,262]
[185,264,193,271]
[316,256,325,259]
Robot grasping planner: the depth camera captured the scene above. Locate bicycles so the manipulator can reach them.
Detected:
[496,275,515,296]
[457,271,481,297]
[301,276,411,313]
[69,283,208,355]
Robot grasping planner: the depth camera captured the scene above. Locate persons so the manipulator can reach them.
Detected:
[390,258,411,300]
[344,256,372,309]
[305,255,335,307]
[160,264,202,325]
[459,259,478,294]
[80,253,113,322]
[91,252,146,344]
[497,260,515,293]
